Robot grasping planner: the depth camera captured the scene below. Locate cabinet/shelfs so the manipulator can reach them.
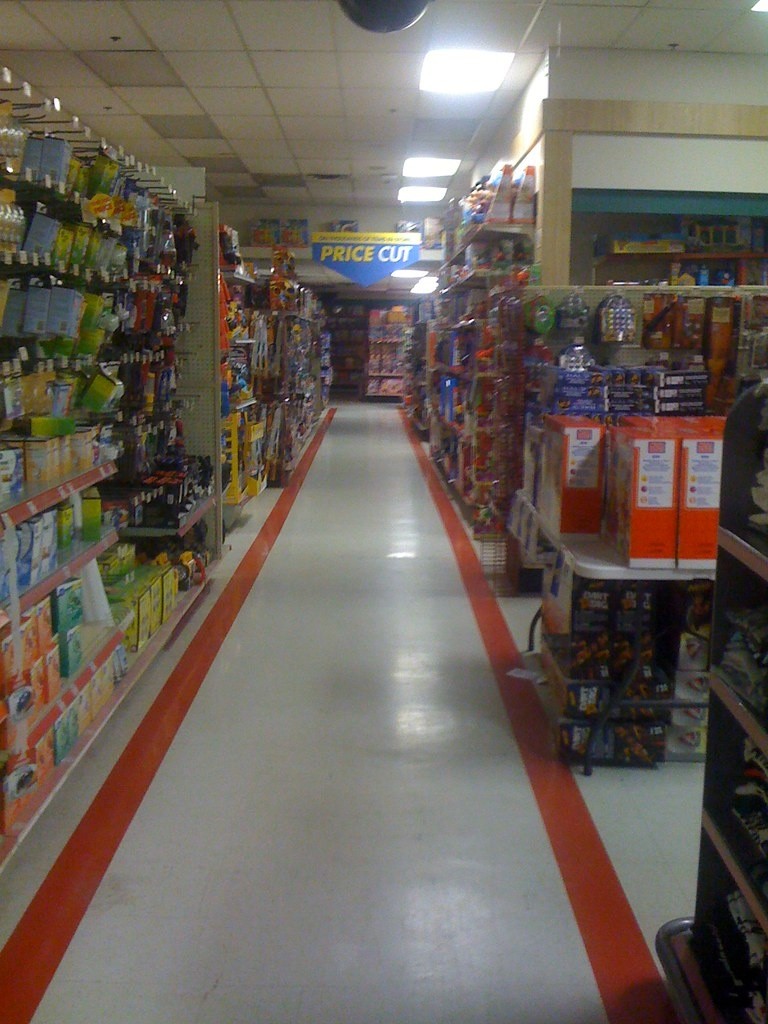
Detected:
[655,378,768,1024]
[362,314,408,404]
[410,319,437,431]
[324,313,363,386]
[416,223,534,508]
[0,463,218,874]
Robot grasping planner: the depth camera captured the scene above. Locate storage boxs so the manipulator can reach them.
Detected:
[1,488,197,838]
[541,553,714,765]
[610,240,686,254]
[0,134,127,497]
[525,364,725,570]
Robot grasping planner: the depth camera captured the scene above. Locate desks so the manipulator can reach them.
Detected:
[515,489,715,778]
